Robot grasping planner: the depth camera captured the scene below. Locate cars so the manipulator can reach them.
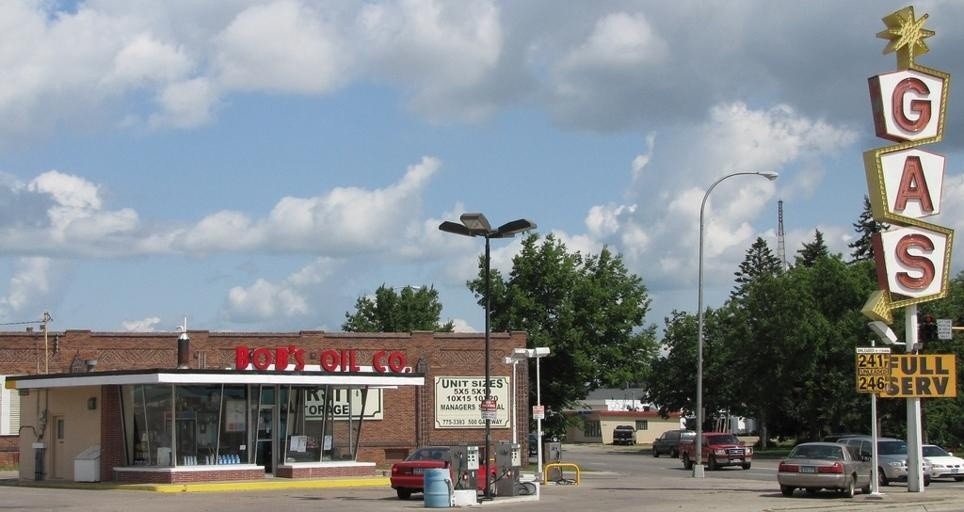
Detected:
[613,425,636,446]
[390,444,496,501]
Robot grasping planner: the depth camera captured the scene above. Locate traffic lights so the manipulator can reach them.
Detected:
[922,315,933,340]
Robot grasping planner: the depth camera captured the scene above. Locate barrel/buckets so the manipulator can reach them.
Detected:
[423,466,453,507]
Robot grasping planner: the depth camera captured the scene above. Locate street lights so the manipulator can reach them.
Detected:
[688,171,780,479]
[867,320,924,494]
[502,347,551,485]
[437,211,537,502]
